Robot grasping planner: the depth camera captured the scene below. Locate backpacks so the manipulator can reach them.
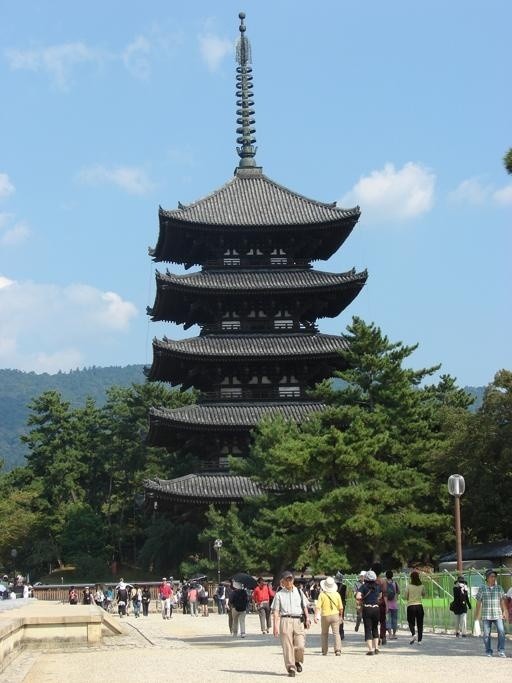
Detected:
[235,590,247,612]
[386,581,395,601]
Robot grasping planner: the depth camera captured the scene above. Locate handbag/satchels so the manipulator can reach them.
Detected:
[304,614,307,629]
[270,598,273,606]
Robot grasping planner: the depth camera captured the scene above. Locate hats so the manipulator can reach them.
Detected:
[358,570,377,581]
[319,576,338,592]
[485,569,498,579]
[281,570,294,579]
[454,576,468,585]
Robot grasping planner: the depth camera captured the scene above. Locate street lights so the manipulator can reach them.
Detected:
[10,546,18,591]
[446,471,467,578]
[213,537,224,582]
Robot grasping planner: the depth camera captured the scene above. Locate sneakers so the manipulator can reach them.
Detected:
[241,633,245,638]
[233,634,237,638]
[118,610,228,620]
[279,628,506,677]
[263,628,269,634]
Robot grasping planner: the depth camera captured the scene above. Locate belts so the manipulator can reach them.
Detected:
[281,614,301,619]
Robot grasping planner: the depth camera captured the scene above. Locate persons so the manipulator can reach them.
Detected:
[269,570,312,677]
